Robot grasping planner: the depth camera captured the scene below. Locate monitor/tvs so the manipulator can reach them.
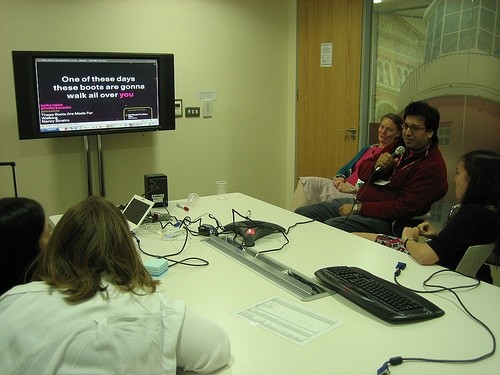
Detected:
[11,50,176,140]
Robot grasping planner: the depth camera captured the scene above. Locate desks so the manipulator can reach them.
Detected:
[49,192,500,375]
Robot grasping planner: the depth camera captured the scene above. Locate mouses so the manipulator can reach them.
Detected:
[198,224,219,237]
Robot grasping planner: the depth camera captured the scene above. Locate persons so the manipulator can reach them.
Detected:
[351,149,500,272]
[294,101,448,238]
[0,195,231,375]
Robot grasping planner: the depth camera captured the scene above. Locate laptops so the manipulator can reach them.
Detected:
[122,194,155,234]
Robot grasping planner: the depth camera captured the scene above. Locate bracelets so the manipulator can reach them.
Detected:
[404,237,418,248]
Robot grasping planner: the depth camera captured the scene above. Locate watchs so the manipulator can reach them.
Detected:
[353,203,358,214]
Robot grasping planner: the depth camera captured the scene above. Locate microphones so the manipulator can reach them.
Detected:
[373,146,406,173]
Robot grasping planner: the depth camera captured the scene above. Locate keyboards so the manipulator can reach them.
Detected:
[314,266,446,322]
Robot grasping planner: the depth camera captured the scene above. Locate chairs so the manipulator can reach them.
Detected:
[455,243,496,279]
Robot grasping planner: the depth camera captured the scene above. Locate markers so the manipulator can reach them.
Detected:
[176,203,189,211]
[245,209,251,219]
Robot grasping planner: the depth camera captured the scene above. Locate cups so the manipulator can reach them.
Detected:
[187,193,199,208]
[216,180,227,199]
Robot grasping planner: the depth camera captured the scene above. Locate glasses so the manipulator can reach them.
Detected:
[400,122,426,134]
